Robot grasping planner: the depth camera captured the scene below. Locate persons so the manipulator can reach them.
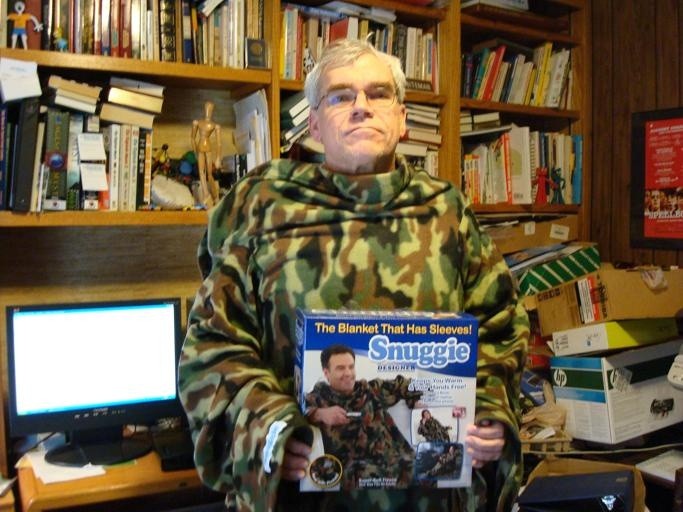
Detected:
[177,35,534,512]
[643,187,683,218]
[416,409,451,443]
[428,444,457,477]
[303,342,423,487]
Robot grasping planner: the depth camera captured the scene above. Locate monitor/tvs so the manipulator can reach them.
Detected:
[5,297,182,468]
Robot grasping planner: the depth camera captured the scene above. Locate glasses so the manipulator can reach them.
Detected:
[314,86,400,110]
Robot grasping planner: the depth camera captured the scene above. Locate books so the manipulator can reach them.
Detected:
[504,243,583,277]
[459,1,581,207]
[279,1,442,179]
[1,1,274,212]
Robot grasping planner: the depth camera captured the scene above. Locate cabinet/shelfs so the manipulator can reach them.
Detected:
[0,0,592,405]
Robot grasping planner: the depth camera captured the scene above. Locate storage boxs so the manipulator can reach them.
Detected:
[535,266,682,446]
